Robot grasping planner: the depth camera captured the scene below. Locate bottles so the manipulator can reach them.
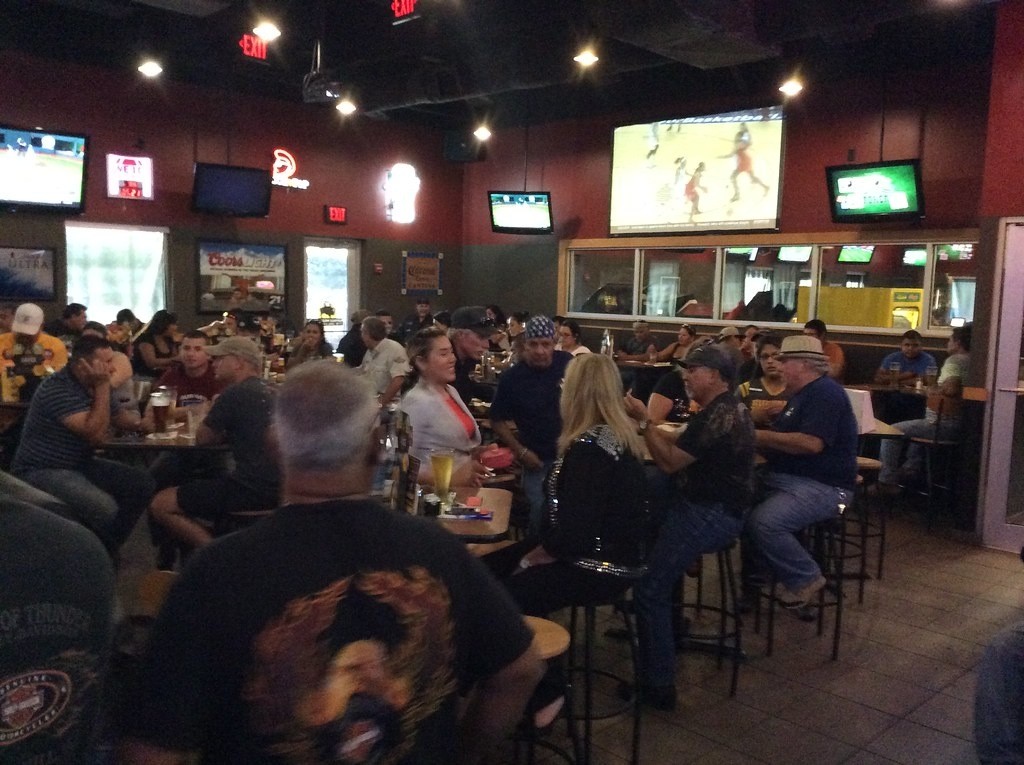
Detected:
[275,358,286,383]
[915,378,926,397]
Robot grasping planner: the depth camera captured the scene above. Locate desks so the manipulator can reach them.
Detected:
[100,358,927,572]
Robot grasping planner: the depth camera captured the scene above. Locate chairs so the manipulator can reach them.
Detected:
[895,386,988,516]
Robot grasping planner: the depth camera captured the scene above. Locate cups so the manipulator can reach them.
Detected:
[187,409,205,437]
[430,448,455,504]
[927,366,938,390]
[151,393,171,439]
[332,353,344,365]
[889,362,900,388]
[650,351,657,364]
[482,356,495,380]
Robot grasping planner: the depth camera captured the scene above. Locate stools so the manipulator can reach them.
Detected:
[514,456,886,765]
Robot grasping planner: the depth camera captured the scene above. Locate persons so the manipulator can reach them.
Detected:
[113,358,543,764]
[645,105,770,221]
[0,299,336,764]
[339,296,973,724]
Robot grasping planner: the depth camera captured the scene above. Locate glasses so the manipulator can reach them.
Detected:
[760,353,778,360]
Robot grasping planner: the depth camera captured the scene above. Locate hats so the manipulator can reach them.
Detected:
[719,327,746,341]
[451,306,501,336]
[203,336,261,366]
[678,347,728,372]
[12,303,44,335]
[772,336,830,361]
[525,316,556,337]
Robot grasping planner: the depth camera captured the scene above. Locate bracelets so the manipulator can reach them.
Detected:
[518,447,528,460]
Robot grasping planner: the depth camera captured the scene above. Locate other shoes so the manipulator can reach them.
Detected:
[619,681,678,710]
[780,577,826,609]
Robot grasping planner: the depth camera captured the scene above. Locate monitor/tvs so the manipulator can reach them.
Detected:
[190,163,272,219]
[902,248,927,267]
[837,245,876,264]
[825,159,926,223]
[937,244,974,261]
[725,248,758,262]
[777,246,812,263]
[0,124,90,214]
[488,191,554,234]
